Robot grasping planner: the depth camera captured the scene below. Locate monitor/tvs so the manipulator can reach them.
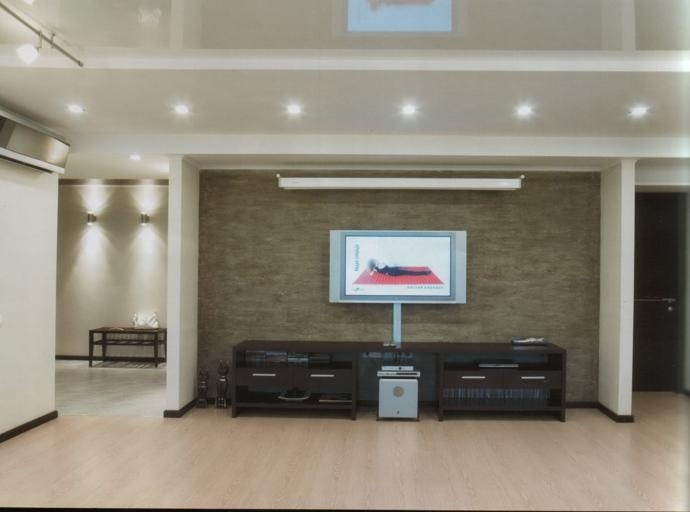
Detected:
[329,229,467,304]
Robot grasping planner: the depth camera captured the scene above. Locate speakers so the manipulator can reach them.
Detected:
[378,378,418,419]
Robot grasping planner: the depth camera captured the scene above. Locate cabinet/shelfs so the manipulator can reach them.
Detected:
[89,326,168,369]
[229,345,359,421]
[439,352,567,422]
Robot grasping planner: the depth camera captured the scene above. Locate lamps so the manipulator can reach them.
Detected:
[140,212,149,225]
[86,211,97,225]
[17,32,44,69]
